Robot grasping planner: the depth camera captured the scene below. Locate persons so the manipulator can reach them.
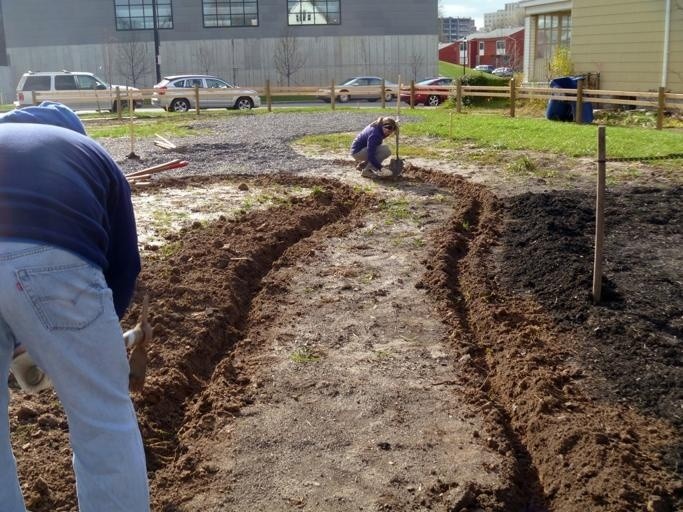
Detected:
[1,99,150,512]
[350,116,398,179]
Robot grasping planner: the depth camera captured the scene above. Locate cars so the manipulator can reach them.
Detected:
[314,76,398,104]
[491,67,514,78]
[472,65,495,73]
[398,76,463,109]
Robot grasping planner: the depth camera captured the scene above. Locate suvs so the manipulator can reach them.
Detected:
[12,72,142,114]
[151,73,263,114]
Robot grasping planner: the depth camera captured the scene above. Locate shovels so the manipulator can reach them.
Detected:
[126,88,139,160]
[390,75,403,178]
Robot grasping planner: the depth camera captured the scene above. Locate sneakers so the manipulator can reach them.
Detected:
[355,162,380,179]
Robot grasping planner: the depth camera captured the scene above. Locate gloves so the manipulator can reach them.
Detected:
[10,353,54,395]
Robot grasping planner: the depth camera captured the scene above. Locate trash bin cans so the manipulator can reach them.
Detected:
[546,76,593,123]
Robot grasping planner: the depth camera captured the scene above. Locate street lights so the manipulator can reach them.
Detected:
[460,37,467,78]
[503,34,517,69]
